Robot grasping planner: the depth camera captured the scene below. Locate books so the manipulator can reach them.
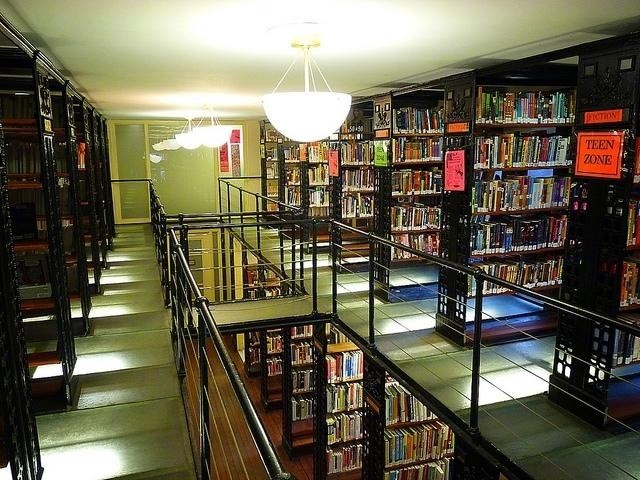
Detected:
[326,321,364,477]
[244,266,261,367]
[306,130,331,218]
[592,131,640,370]
[384,369,455,480]
[464,83,577,298]
[390,104,446,260]
[282,134,303,212]
[290,324,317,421]
[263,266,284,377]
[339,105,377,219]
[265,121,280,213]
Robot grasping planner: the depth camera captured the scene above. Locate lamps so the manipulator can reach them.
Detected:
[175,114,201,150]
[194,105,233,151]
[152,131,180,152]
[260,34,352,142]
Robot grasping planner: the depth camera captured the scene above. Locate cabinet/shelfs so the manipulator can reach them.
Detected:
[281,280,314,461]
[240,244,263,377]
[0,62,117,480]
[451,434,509,480]
[311,318,365,480]
[361,354,457,480]
